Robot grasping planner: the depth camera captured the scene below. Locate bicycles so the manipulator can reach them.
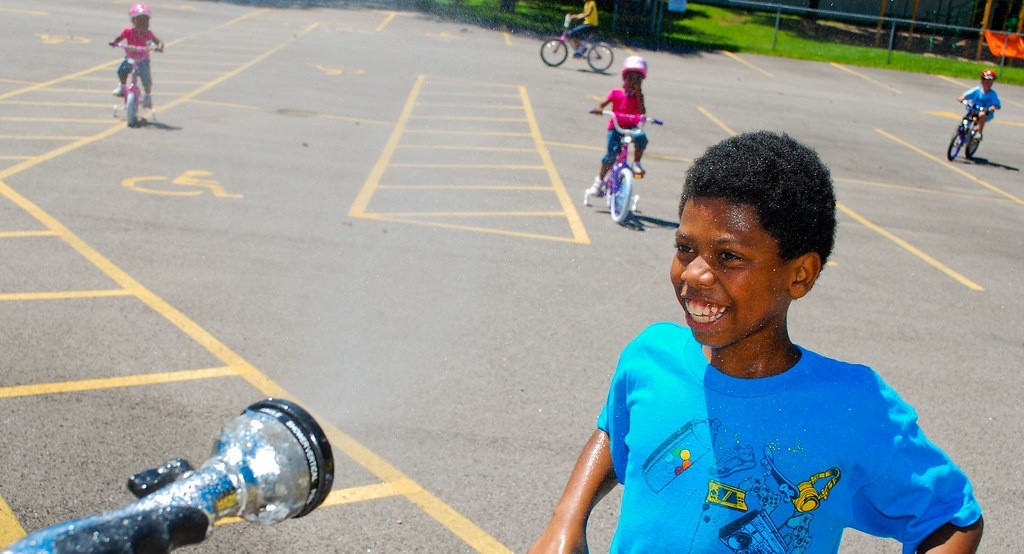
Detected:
[539,14,616,73]
[946,98,991,162]
[109,43,163,127]
[584,108,665,224]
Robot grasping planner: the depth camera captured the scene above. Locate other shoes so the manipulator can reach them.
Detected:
[573,47,587,58]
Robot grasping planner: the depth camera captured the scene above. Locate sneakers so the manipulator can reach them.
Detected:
[113,86,124,95]
[973,133,982,140]
[594,177,605,196]
[144,95,151,106]
[631,162,645,174]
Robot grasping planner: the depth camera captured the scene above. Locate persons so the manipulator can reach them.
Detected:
[957,68,1001,139]
[564,0,598,58]
[527,129,985,554]
[584,55,649,196]
[110,3,163,108]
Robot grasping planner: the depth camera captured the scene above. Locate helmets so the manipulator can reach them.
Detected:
[621,56,647,81]
[129,3,151,18]
[981,69,996,81]
